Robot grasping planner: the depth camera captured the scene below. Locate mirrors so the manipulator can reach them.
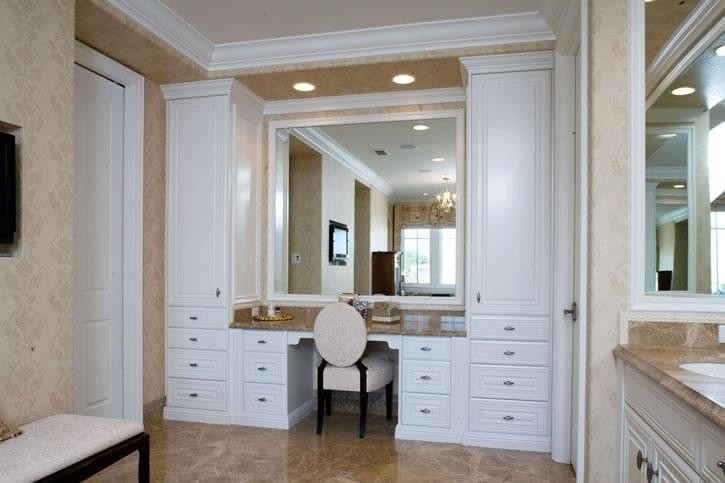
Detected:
[267,108,464,307]
[628,0,725,313]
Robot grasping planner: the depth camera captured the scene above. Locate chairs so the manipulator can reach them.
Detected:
[313,302,394,438]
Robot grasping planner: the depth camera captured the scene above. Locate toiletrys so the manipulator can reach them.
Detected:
[275,306,282,317]
[267,302,274,316]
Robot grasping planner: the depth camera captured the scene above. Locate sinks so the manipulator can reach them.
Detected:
[678,358,725,380]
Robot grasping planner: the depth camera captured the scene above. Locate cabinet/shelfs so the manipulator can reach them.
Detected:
[372,252,401,296]
[468,69,552,454]
[399,335,451,429]
[163,96,230,424]
[695,413,725,483]
[622,361,695,483]
[242,329,285,419]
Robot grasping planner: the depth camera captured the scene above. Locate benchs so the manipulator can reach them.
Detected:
[0,413,150,483]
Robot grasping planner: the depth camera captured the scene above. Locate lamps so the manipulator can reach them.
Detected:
[434,178,456,211]
